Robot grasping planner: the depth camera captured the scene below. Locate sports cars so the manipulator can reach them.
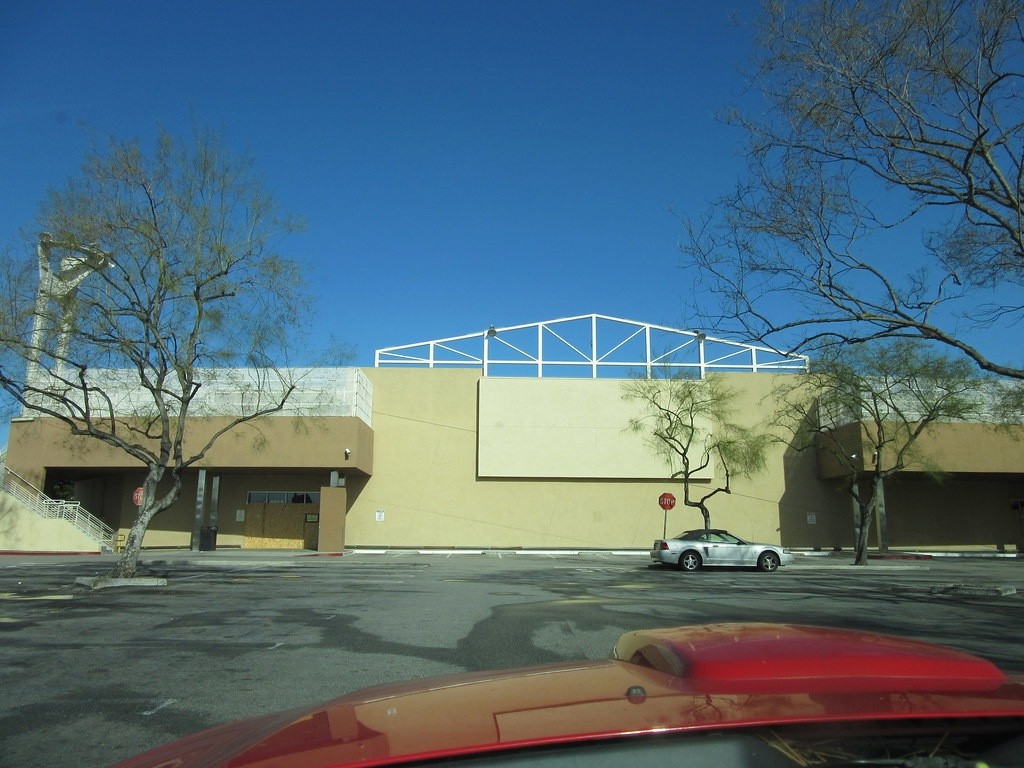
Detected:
[650,529,796,573]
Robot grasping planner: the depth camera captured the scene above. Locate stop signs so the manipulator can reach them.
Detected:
[659,493,676,510]
[133,488,144,506]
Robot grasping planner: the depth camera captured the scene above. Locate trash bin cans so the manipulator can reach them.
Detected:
[190,526,217,551]
